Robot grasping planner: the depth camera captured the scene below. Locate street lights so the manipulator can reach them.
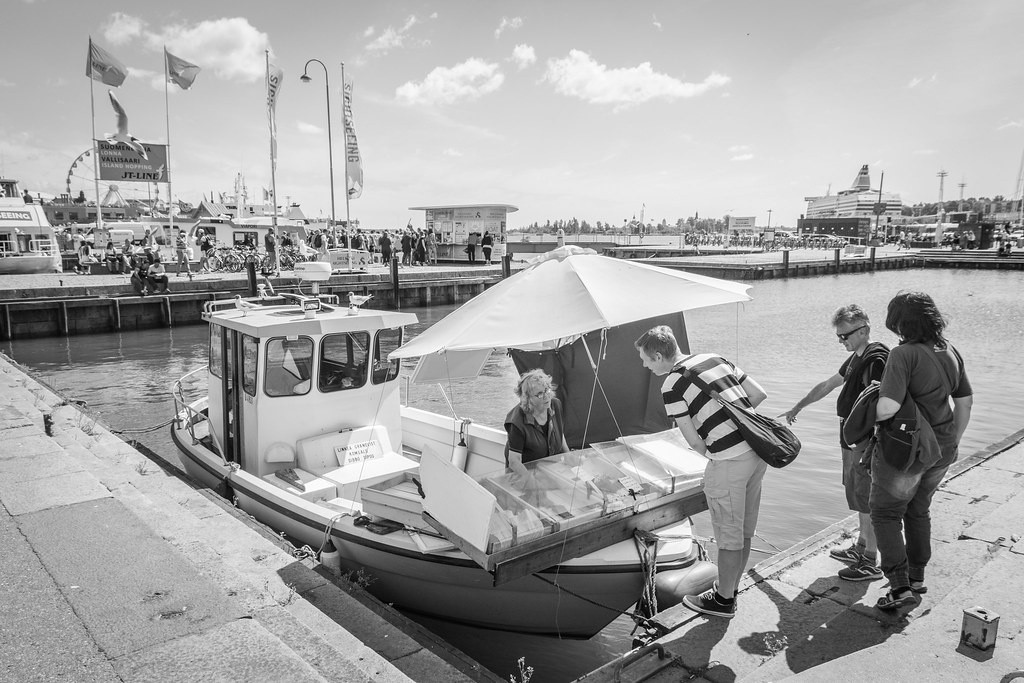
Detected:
[300,58,338,249]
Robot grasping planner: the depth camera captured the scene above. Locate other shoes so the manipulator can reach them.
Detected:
[128,265,132,268]
[165,289,170,293]
[140,290,148,294]
[188,272,195,277]
[121,271,125,275]
[153,290,160,293]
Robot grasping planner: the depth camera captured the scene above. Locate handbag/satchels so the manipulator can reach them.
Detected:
[722,402,801,468]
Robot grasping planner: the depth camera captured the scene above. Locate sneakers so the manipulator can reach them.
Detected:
[838,555,884,580]
[682,592,735,618]
[830,544,864,563]
[713,580,738,610]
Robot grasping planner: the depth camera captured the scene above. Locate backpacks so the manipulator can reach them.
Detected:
[315,234,324,248]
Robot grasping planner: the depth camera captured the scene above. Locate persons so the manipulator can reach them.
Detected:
[175,230,195,277]
[23,189,33,203]
[635,325,768,618]
[777,304,891,581]
[505,368,570,492]
[482,232,492,265]
[868,291,974,608]
[1005,222,1012,235]
[946,231,978,250]
[998,242,1011,254]
[265,227,276,272]
[380,228,439,268]
[280,231,293,246]
[195,229,214,252]
[897,229,912,250]
[467,233,478,264]
[61,229,170,295]
[309,229,377,264]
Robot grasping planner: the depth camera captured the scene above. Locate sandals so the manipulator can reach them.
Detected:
[877,586,917,609]
[910,580,927,593]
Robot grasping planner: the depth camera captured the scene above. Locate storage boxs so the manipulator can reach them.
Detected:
[362,471,440,534]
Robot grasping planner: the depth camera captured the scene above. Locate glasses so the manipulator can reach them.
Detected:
[530,386,551,399]
[836,325,868,340]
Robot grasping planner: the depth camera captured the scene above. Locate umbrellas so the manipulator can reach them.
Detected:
[387,228,754,362]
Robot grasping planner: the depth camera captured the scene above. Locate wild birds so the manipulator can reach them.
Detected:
[103,89,149,161]
[233,294,261,317]
[347,292,374,308]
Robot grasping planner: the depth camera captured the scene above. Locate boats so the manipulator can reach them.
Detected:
[0,172,334,275]
[170,248,753,640]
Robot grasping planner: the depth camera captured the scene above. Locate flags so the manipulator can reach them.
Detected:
[86,41,129,87]
[166,51,200,90]
